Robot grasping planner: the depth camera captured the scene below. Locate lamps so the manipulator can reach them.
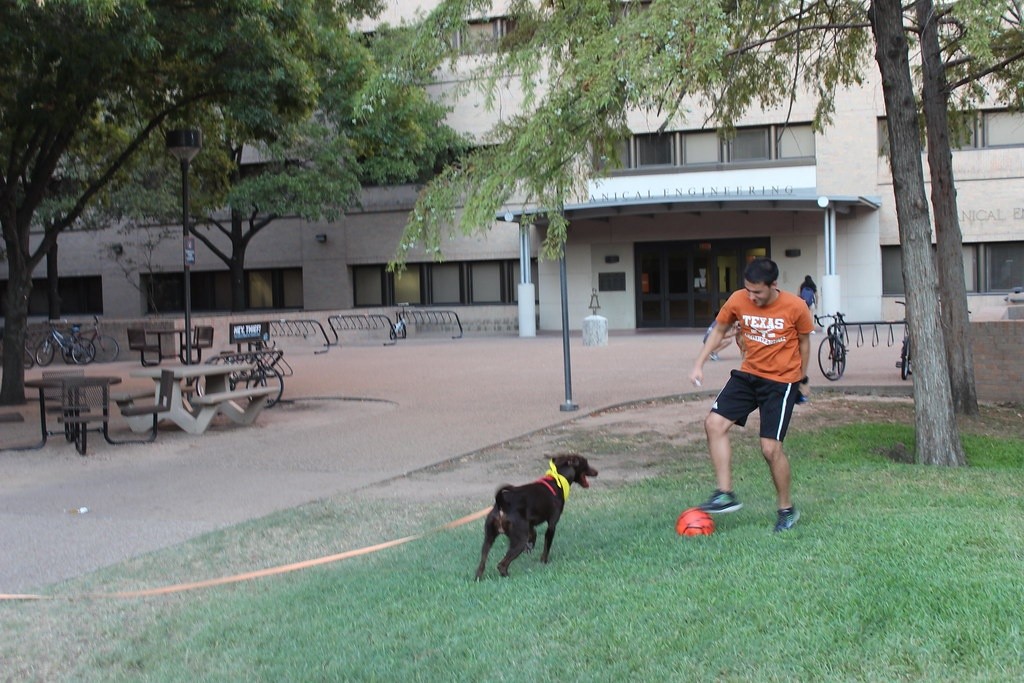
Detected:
[315,233,326,242]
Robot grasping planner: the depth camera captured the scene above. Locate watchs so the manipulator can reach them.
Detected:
[799,375,810,385]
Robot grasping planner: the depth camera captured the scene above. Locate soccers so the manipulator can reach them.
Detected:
[675,506,716,537]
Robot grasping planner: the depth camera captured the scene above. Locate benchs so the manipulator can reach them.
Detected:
[110,386,195,402]
[191,387,282,405]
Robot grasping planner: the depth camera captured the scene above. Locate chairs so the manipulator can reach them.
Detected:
[127,327,157,352]
[182,325,214,349]
[42,369,87,415]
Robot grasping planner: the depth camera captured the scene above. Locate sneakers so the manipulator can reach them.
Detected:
[772,506,800,533]
[700,488,743,516]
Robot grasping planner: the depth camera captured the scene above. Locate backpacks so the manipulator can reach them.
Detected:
[801,286,814,306]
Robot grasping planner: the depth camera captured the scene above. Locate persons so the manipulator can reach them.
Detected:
[796,275,819,336]
[690,258,816,533]
[702,310,738,361]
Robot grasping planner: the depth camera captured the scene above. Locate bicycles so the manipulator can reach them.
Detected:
[895,301,912,380]
[195,340,284,409]
[25,316,119,369]
[813,312,848,380]
[388,304,408,339]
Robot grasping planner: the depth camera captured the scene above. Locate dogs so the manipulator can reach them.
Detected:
[472,452,599,583]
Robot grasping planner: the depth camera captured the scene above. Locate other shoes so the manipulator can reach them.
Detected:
[709,352,720,360]
[809,330,816,334]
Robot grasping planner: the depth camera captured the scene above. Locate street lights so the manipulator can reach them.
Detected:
[166,130,202,402]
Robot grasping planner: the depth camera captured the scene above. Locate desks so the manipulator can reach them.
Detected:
[126,364,256,433]
[23,376,124,451]
[145,329,200,365]
